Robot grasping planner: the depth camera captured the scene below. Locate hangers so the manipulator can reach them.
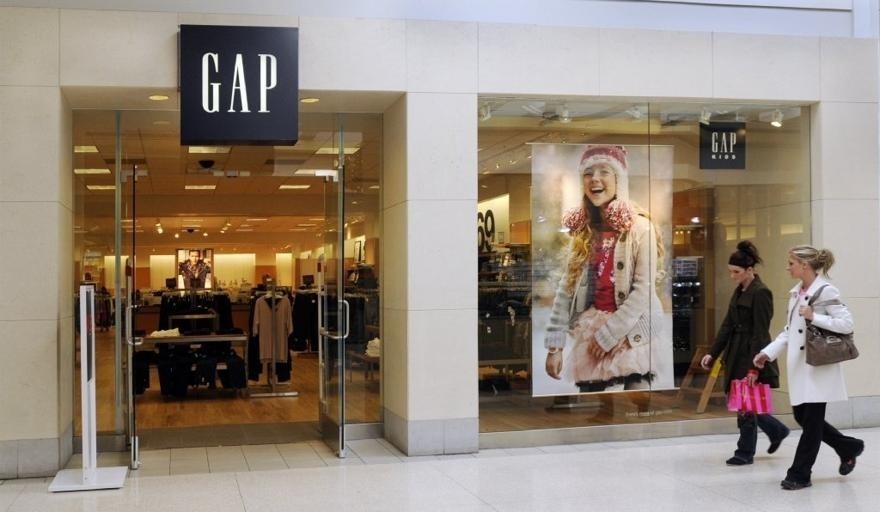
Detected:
[94,281,532,304]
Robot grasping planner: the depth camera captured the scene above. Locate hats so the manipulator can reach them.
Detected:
[579,143,630,200]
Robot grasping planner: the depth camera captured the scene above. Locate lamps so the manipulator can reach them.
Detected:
[479,98,785,128]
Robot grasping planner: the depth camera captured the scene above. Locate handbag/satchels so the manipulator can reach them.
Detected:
[726,371,775,415]
[805,284,859,366]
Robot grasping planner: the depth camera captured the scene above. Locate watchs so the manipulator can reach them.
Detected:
[548,347,564,353]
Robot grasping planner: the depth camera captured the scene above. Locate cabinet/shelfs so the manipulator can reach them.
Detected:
[123,331,251,404]
[479,315,533,407]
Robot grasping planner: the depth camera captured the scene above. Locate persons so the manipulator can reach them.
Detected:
[698,238,790,468]
[176,248,212,287]
[542,145,665,395]
[752,244,866,492]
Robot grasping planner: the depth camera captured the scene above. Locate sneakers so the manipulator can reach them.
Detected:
[726,456,747,466]
[839,439,864,475]
[781,476,811,489]
[766,440,781,453]
